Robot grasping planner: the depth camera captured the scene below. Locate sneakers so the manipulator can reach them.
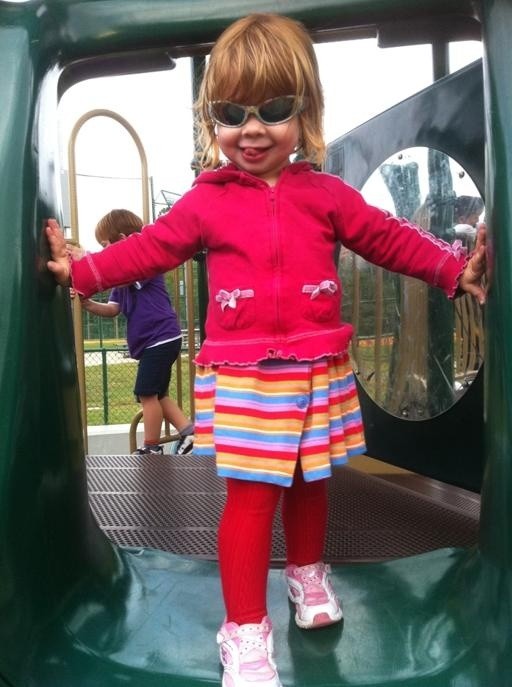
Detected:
[286,560,343,630]
[216,616,282,687]
[131,444,163,455]
[175,433,194,455]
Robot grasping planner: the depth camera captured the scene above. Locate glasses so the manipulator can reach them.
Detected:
[207,94,309,127]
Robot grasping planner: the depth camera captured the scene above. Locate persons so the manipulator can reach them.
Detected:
[451,196,484,242]
[68,208,195,456]
[47,10,487,687]
